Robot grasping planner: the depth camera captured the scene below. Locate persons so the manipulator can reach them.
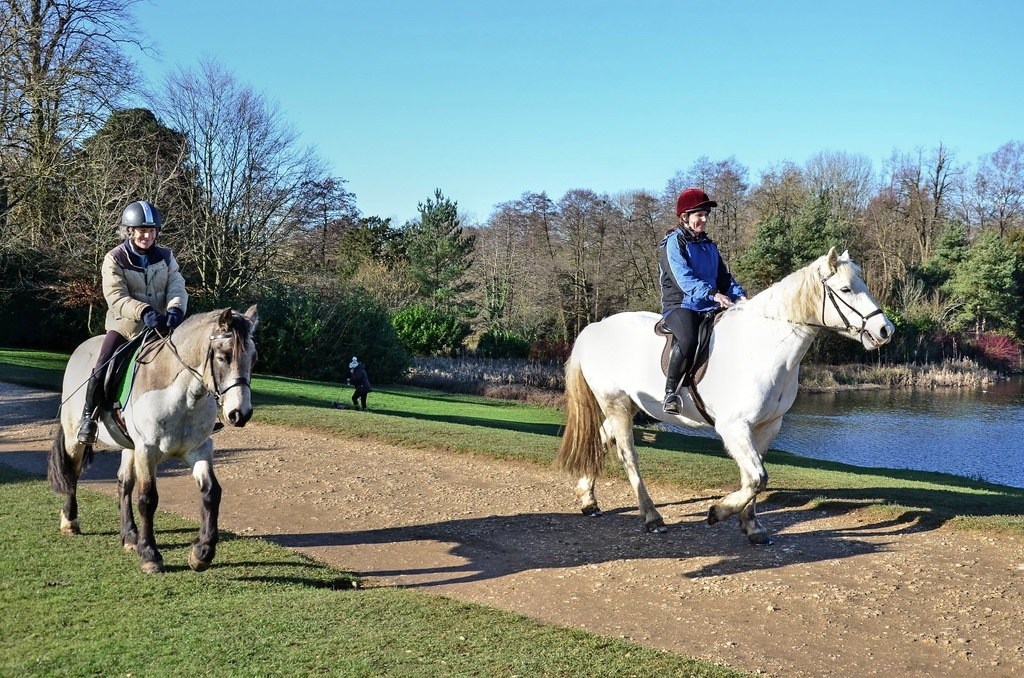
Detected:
[657,189,748,415]
[77,200,225,445]
[346,357,372,411]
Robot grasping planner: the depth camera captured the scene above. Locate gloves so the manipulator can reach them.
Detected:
[143,310,166,330]
[166,308,184,329]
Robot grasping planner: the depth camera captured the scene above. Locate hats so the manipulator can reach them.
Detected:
[349,357,359,368]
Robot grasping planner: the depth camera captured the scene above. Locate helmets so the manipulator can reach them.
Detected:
[677,189,717,225]
[121,201,161,233]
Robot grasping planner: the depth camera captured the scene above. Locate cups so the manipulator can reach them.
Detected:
[347,379,350,385]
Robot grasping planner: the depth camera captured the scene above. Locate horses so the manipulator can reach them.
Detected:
[551,245,896,547]
[46,303,260,575]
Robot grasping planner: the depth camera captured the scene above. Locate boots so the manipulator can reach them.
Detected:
[664,345,687,413]
[78,368,105,441]
